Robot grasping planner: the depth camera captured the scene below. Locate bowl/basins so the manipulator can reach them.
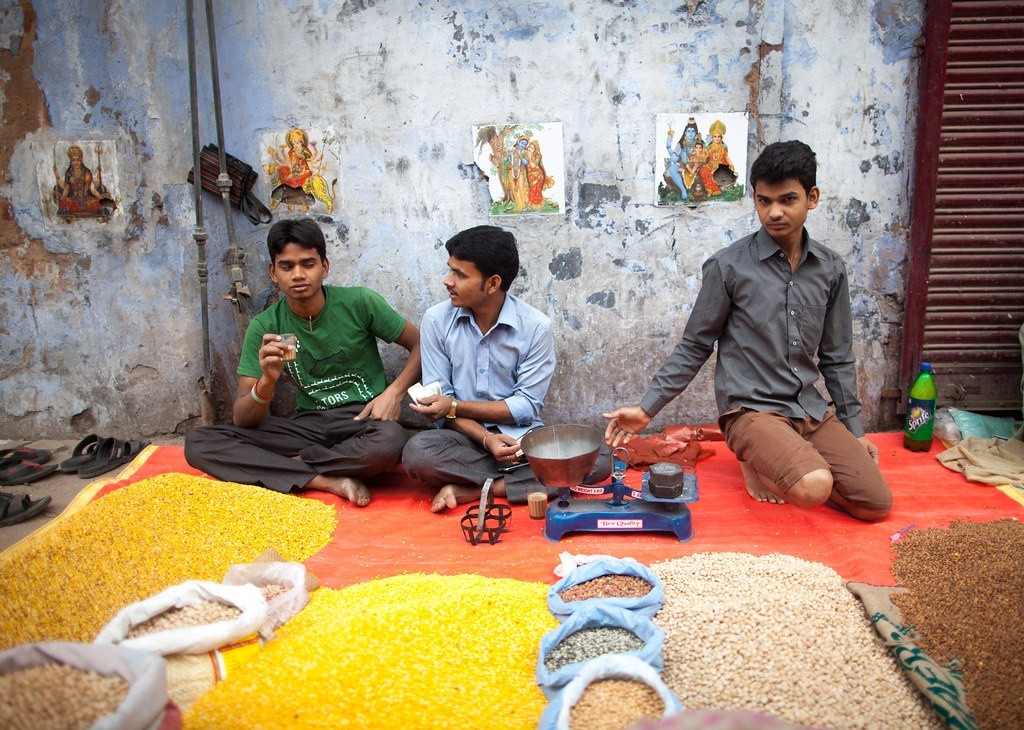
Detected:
[521,424,604,488]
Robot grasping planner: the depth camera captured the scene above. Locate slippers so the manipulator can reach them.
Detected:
[0,448,58,486]
[60,434,151,479]
[0,491,52,527]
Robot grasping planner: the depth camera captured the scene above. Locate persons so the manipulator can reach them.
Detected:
[603,141,892,522]
[183,218,420,507]
[402,226,614,513]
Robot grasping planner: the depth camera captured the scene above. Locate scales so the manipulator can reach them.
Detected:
[520,424,699,543]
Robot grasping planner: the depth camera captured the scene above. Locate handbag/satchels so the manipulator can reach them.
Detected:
[187,142,272,225]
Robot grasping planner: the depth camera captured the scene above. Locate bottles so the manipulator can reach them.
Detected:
[904,362,937,452]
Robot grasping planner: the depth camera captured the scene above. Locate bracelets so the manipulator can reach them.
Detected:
[446,400,458,419]
[249,381,275,405]
[481,432,495,448]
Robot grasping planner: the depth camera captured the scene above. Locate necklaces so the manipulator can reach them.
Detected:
[286,299,327,332]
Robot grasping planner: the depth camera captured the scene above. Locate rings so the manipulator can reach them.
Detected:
[507,456,510,460]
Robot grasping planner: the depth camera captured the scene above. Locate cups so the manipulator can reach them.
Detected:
[526,486,548,521]
[279,333,296,362]
